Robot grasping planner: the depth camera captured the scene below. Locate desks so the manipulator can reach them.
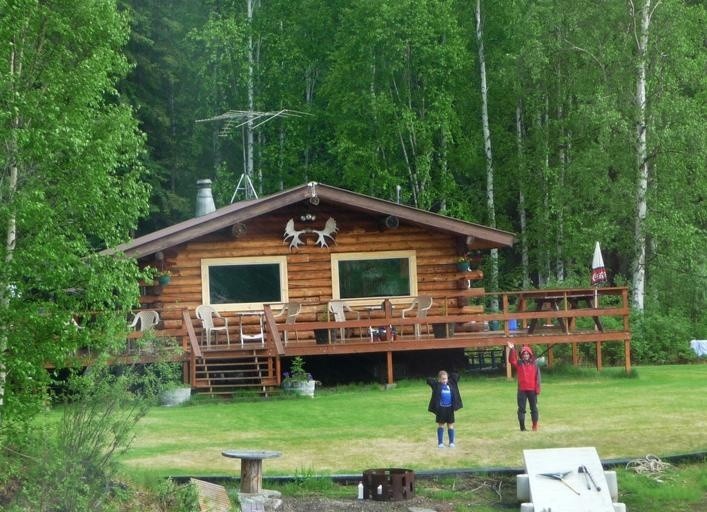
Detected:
[527,294,603,335]
[236,312,266,349]
[364,305,397,342]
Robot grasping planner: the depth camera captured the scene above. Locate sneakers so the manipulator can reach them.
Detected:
[437,442,454,448]
[519,425,537,431]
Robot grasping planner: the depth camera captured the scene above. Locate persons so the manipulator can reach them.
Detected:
[507,341,541,432]
[426,370,463,448]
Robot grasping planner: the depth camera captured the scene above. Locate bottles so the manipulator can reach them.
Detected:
[357,481,364,499]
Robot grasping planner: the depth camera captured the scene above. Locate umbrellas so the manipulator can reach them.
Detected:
[591,241,607,330]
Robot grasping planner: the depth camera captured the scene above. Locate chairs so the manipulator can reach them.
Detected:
[273,301,302,346]
[326,299,364,342]
[195,305,230,348]
[127,309,160,355]
[399,295,432,339]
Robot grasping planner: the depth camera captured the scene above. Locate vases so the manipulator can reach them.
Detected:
[285,380,315,399]
[160,383,191,408]
